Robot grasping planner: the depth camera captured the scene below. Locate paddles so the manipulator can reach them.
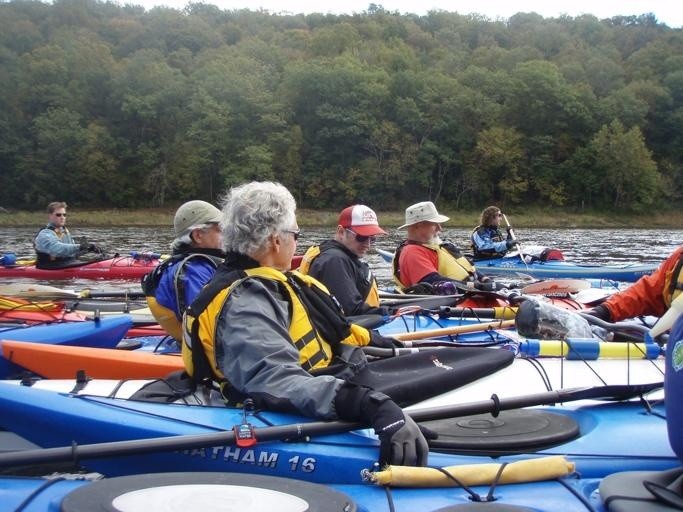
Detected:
[20,283,145,299]
[397,337,511,349]
[0,381,665,470]
[450,280,619,331]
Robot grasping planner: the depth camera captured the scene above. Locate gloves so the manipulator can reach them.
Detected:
[479,281,509,291]
[511,239,521,247]
[506,225,514,237]
[581,305,611,326]
[87,242,105,254]
[372,328,407,350]
[362,390,439,472]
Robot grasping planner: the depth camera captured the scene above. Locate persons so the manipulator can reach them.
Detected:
[587,245,683,325]
[141,200,225,340]
[471,205,521,257]
[34,201,102,270]
[299,204,421,314]
[182,180,439,467]
[391,201,510,294]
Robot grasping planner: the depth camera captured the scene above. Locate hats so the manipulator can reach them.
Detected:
[397,201,451,230]
[337,204,389,238]
[173,199,223,237]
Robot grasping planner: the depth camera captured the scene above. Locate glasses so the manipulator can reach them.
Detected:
[346,228,377,244]
[52,213,66,218]
[497,214,503,217]
[285,228,301,242]
